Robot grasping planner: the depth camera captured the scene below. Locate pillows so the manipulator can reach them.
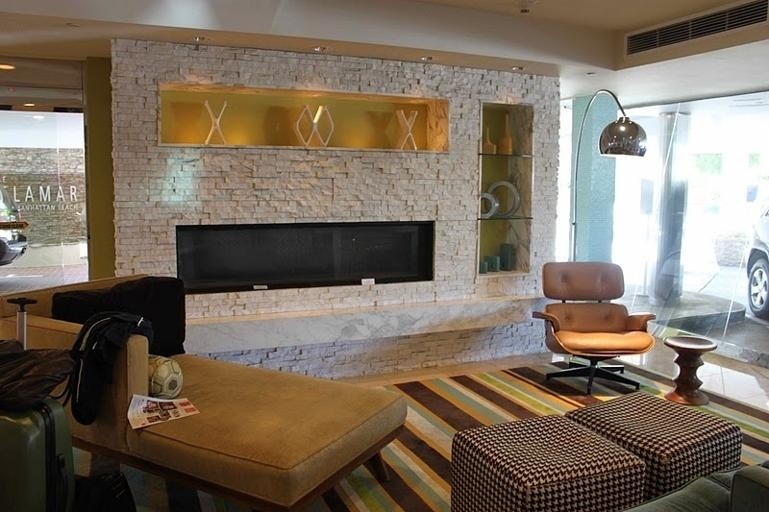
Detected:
[147,354,183,401]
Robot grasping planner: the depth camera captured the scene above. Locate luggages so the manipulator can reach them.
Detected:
[0,298,77,512]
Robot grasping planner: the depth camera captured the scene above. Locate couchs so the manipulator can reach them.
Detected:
[0,274,408,512]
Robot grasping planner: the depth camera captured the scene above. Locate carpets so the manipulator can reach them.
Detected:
[72,358,769,512]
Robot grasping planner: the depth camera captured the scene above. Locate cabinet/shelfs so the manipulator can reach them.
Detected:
[478,152,533,220]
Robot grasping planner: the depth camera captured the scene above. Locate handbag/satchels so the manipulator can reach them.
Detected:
[1,339,76,413]
[75,471,136,512]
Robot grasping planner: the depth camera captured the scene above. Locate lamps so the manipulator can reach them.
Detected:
[573,89,647,261]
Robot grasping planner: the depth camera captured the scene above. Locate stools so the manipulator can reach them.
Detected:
[450,414,646,512]
[565,390,743,504]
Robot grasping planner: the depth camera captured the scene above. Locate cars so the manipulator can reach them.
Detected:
[743,207,769,318]
[0,191,29,266]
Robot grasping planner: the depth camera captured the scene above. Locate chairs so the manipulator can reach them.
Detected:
[619,460,769,512]
[532,262,656,394]
